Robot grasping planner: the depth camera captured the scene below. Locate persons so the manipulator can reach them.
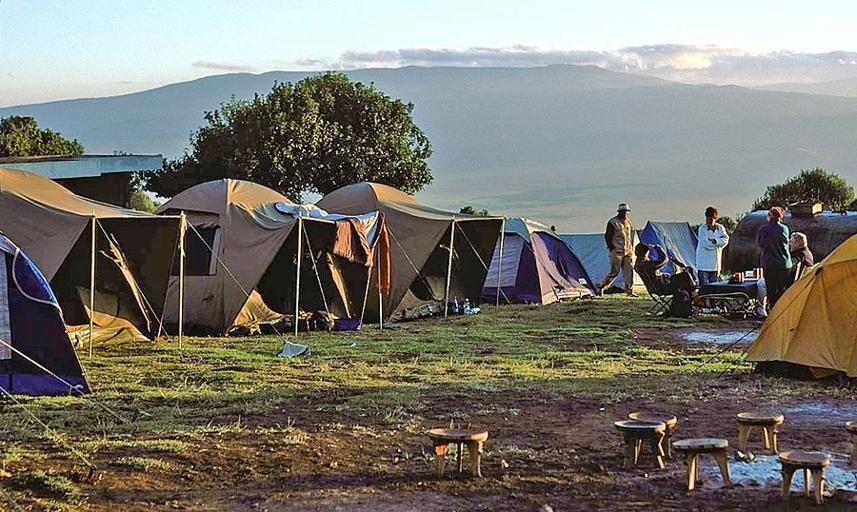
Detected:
[755,206,792,308]
[594,203,639,297]
[634,242,700,305]
[695,207,729,313]
[752,232,814,318]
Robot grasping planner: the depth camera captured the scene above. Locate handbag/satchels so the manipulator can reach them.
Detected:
[671,289,690,319]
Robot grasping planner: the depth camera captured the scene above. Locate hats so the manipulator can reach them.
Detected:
[617,204,631,212]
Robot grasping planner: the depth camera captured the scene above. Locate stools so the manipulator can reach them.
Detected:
[615,421,668,470]
[428,428,490,479]
[630,412,677,457]
[673,438,734,492]
[779,451,829,505]
[737,412,785,456]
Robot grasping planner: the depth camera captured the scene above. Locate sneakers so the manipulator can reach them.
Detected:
[595,283,601,297]
[627,293,639,297]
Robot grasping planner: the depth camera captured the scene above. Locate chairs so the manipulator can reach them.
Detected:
[636,269,695,315]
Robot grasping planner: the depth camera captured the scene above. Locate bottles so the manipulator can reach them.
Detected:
[463,299,471,315]
[451,296,459,314]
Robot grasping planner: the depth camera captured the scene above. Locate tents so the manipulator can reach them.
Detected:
[151,178,380,335]
[1,235,93,396]
[313,181,507,323]
[639,221,723,283]
[560,229,646,295]
[746,234,857,390]
[482,217,598,304]
[1,165,185,347]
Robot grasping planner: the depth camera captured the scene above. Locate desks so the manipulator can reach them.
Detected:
[702,280,758,322]
